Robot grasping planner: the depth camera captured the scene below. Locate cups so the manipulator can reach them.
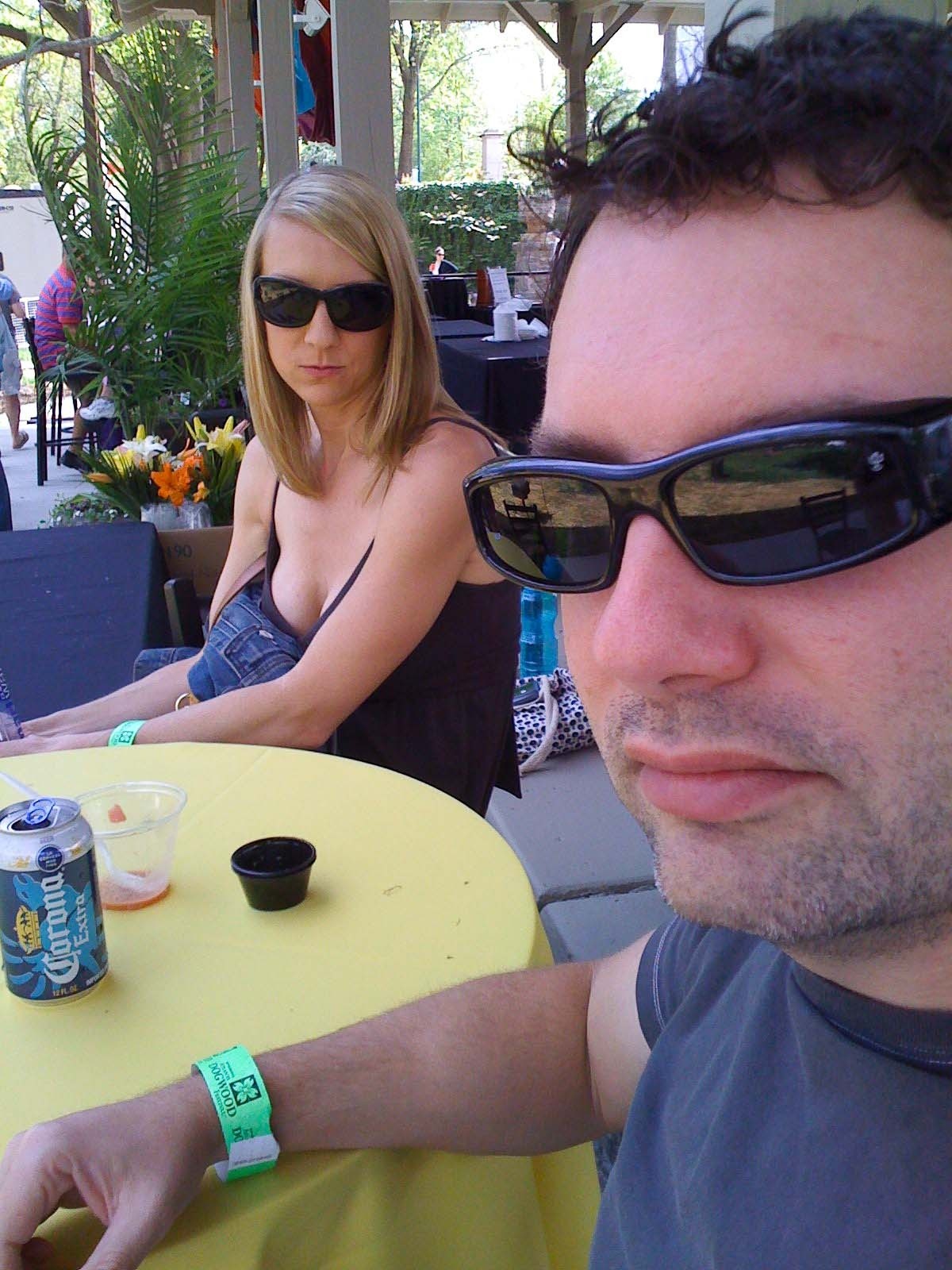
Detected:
[494,311,519,342]
[518,329,536,340]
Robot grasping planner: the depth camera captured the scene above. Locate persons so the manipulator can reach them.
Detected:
[1,7,951,1268]
[0,164,524,818]
[0,252,29,459]
[34,235,196,475]
[429,246,459,275]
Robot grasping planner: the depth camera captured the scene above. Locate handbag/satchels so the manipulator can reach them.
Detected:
[509,668,596,776]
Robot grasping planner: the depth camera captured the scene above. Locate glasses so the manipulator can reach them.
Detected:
[437,253,445,256]
[250,273,394,334]
[463,395,952,595]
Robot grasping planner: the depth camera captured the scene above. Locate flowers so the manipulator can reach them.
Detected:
[63,421,243,528]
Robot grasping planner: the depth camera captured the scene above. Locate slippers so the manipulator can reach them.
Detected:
[13,431,29,449]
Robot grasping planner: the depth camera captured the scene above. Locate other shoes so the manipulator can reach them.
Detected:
[61,449,95,475]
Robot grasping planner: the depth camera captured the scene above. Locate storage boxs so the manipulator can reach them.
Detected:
[154,527,234,600]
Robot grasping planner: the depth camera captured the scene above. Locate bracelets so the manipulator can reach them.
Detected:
[191,1044,280,1183]
[108,719,147,747]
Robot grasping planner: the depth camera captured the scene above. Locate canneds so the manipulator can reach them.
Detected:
[0,796,110,1006]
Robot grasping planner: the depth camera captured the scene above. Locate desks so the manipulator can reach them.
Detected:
[432,320,549,441]
[0,739,604,1270]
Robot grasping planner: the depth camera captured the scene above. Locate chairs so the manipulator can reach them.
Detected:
[24,317,101,485]
[0,522,200,723]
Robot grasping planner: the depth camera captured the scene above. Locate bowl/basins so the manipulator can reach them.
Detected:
[230,837,316,911]
[71,781,188,912]
